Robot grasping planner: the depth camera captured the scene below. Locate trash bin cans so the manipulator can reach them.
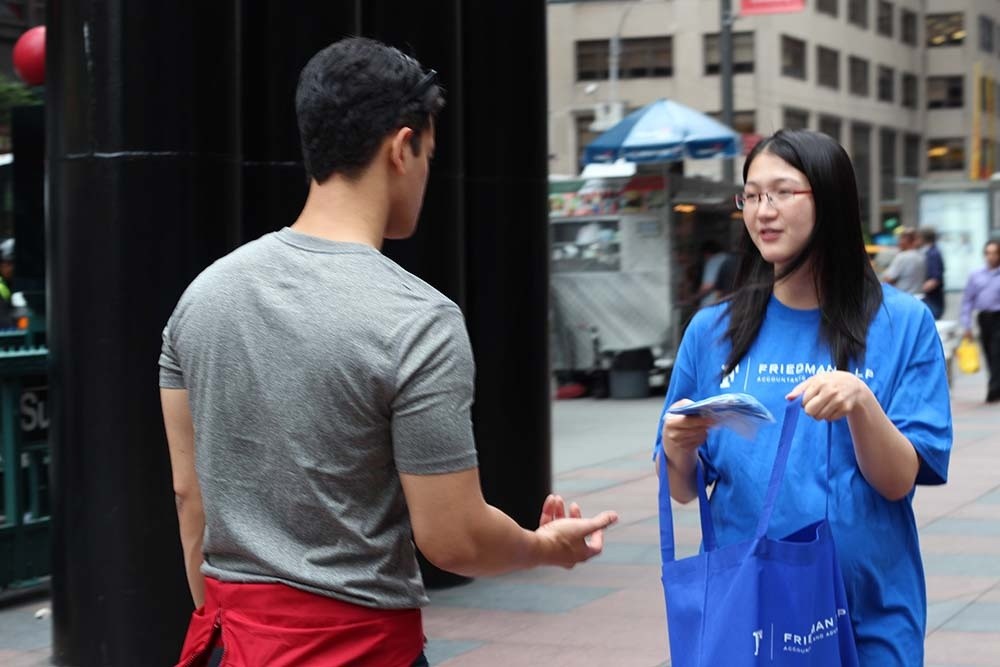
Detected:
[607,346,649,398]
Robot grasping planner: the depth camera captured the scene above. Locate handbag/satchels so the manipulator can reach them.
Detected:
[956,338,981,373]
[655,391,861,667]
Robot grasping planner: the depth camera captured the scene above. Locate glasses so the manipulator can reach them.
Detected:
[733,186,812,211]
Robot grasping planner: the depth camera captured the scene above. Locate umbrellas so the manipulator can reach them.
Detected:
[581,98,739,162]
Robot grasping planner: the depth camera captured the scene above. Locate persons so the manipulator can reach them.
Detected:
[868,224,946,321]
[158,38,619,667]
[960,240,1000,403]
[651,130,954,667]
[0,236,17,305]
[688,240,737,304]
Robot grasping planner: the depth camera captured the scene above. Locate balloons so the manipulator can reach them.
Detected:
[12,25,48,86]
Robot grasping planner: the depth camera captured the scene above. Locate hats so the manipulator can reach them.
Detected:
[0,238,16,263]
